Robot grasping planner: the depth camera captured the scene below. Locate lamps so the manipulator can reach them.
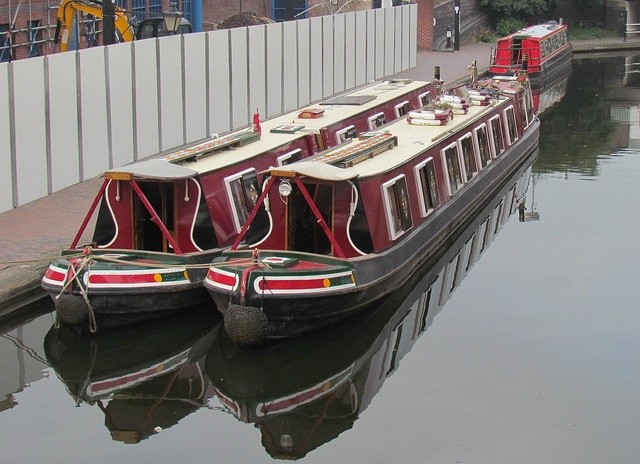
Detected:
[279,179,293,203]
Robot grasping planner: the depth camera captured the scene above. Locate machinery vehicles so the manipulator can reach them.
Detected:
[54,1,193,52]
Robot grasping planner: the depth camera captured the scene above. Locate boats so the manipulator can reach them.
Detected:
[487,17,573,88]
[42,76,440,329]
[204,60,540,344]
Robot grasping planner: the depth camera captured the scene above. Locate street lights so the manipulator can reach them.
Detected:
[160,2,184,35]
